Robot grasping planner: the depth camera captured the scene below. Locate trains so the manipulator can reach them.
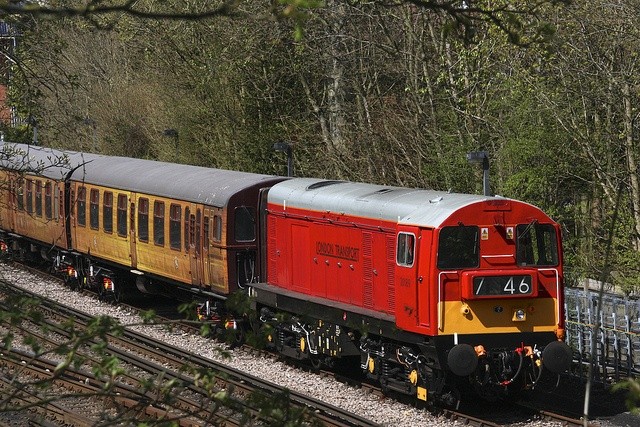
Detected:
[0,140,574,414]
